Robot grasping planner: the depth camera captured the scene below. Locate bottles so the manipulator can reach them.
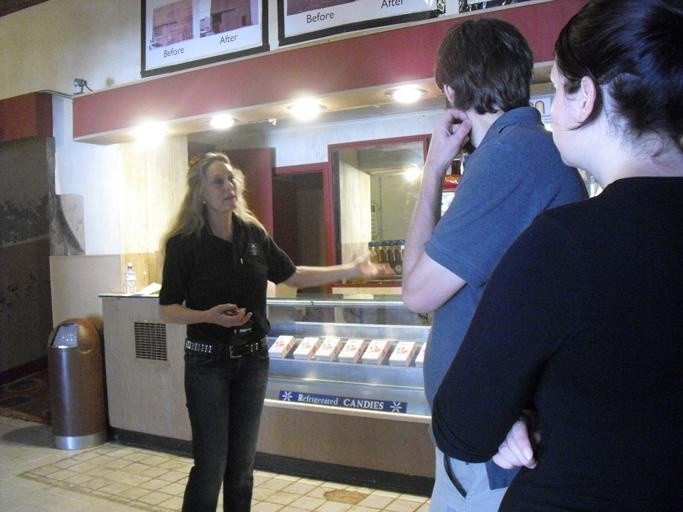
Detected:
[124,262,136,295]
[368,237,405,277]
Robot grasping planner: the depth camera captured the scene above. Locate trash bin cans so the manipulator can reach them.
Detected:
[46,318,107,451]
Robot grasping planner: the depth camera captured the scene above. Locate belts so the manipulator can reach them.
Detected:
[184,339,264,358]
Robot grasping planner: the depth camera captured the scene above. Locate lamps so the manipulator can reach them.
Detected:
[73,78,87,95]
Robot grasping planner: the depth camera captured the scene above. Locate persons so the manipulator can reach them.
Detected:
[429,0,683,508]
[155,153,395,512]
[399,16,593,511]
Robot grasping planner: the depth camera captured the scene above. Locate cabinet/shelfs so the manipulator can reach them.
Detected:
[253,291,437,496]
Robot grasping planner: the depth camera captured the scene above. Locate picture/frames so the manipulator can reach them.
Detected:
[140,0,270,79]
[458,0,532,15]
[277,0,446,48]
